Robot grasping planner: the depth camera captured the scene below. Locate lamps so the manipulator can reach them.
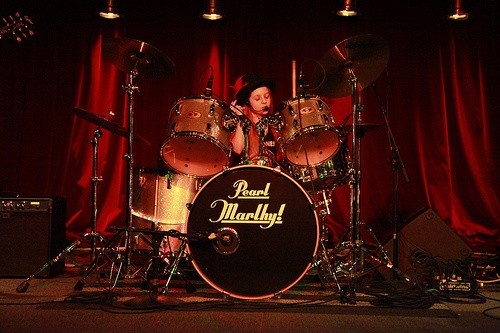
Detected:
[99,0,120,19]
[449,0,468,19]
[202,0,222,20]
[337,0,357,16]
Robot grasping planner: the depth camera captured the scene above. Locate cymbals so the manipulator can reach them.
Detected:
[304,31,391,97]
[75,107,129,138]
[103,37,177,79]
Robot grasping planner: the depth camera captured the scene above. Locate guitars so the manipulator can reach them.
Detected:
[0,12,35,42]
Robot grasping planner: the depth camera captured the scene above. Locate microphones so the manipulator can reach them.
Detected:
[204,78,212,97]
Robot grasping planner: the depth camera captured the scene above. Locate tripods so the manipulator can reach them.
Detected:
[311,54,403,303]
[15,55,197,293]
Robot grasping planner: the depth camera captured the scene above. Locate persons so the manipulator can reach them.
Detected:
[230,75,286,168]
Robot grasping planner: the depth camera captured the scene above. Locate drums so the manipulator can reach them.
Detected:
[183,161,321,301]
[160,93,238,177]
[288,139,354,191]
[131,165,205,224]
[269,90,344,166]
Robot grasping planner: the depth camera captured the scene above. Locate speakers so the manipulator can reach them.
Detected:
[0,198,67,278]
[370,206,474,290]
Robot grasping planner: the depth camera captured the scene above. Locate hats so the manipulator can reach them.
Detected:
[233,73,276,104]
